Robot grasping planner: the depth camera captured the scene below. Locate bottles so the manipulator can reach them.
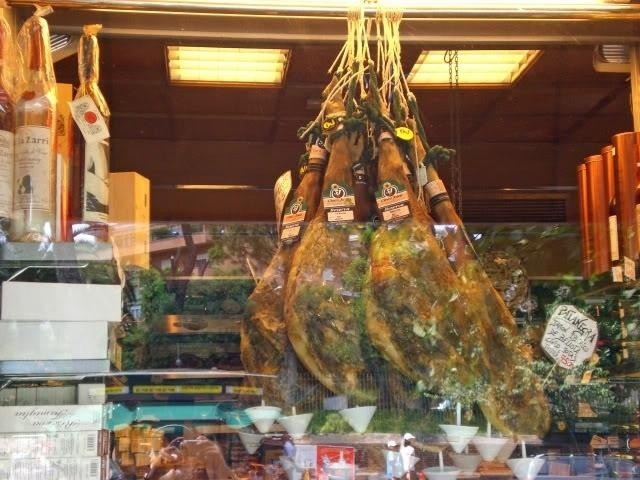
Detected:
[2,5,113,244]
[592,439,640,480]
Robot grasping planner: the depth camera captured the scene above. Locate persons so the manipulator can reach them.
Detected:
[386,439,405,480]
[109,428,296,480]
[398,433,418,479]
